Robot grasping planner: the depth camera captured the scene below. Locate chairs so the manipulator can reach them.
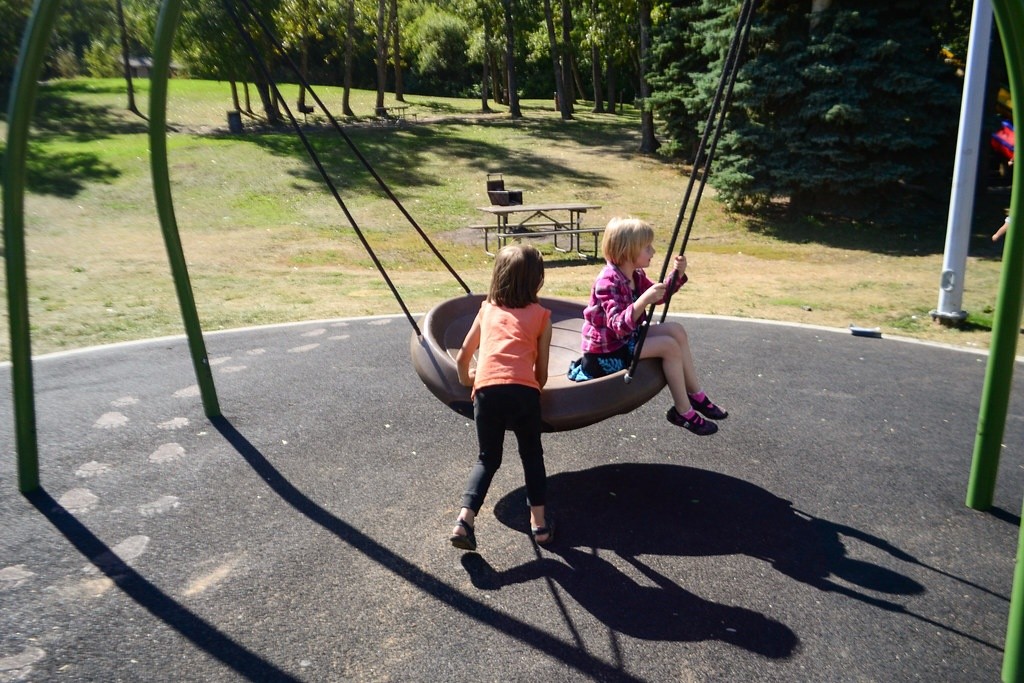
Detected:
[296,98,315,128]
[487,172,523,230]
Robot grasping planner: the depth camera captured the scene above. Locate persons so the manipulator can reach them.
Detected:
[449,244,557,551]
[992,216,1009,243]
[567,218,730,436]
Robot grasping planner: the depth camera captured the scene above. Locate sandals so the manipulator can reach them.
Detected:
[532,510,556,546]
[450,519,477,550]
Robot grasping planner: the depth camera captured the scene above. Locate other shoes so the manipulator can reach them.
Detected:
[689,394,728,420]
[666,406,718,436]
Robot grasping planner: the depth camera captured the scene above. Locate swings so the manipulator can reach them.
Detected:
[222,0,758,433]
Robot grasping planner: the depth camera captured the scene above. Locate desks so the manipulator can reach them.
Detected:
[477,203,602,250]
[373,106,408,127]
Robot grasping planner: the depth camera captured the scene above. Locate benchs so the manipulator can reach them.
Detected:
[467,221,577,257]
[394,112,419,126]
[496,227,605,260]
